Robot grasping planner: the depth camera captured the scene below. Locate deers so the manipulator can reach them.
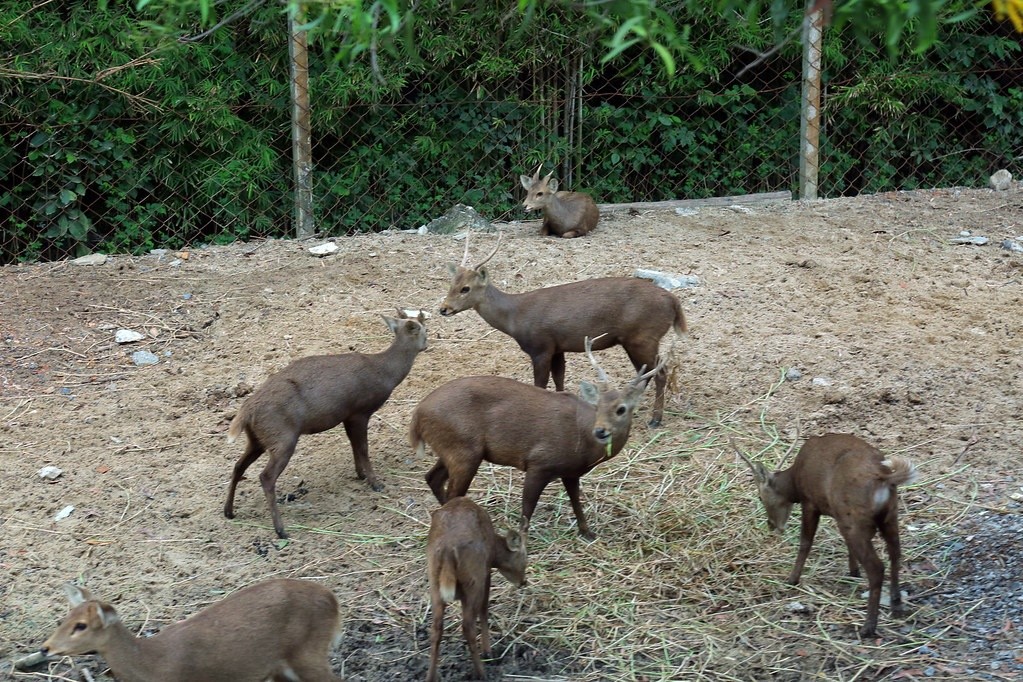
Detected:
[38,163,912,682]
